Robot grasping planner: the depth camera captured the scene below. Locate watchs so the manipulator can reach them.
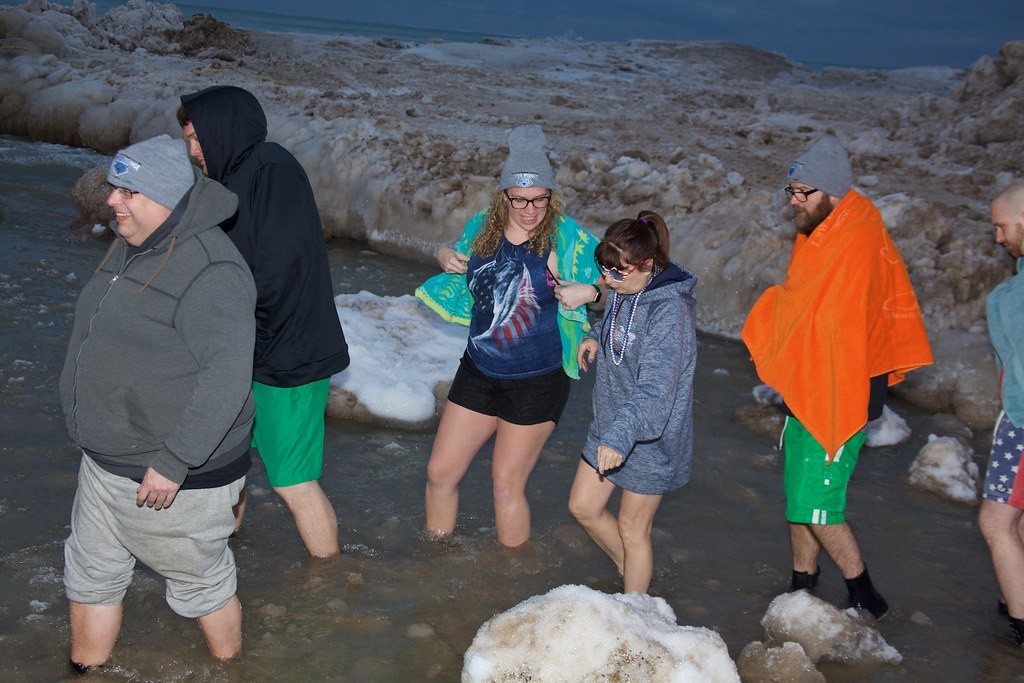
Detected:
[589,283,603,304]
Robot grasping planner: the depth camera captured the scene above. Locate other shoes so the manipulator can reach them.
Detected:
[850,593,890,623]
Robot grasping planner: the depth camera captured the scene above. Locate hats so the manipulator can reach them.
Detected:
[788,135,853,199]
[500,123,555,190]
[106,132,195,213]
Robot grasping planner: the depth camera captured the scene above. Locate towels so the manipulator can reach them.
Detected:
[984,256,1024,430]
[737,188,935,465]
[414,205,606,382]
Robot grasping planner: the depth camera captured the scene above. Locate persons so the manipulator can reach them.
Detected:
[569,210,699,598]
[738,136,932,622]
[55,133,256,683]
[414,122,612,551]
[173,83,356,555]
[974,182,1022,644]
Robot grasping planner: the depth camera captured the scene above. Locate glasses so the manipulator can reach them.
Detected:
[784,187,818,202]
[504,188,552,209]
[103,182,139,199]
[595,259,645,282]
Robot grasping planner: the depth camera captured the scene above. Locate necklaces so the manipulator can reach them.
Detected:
[609,262,657,365]
